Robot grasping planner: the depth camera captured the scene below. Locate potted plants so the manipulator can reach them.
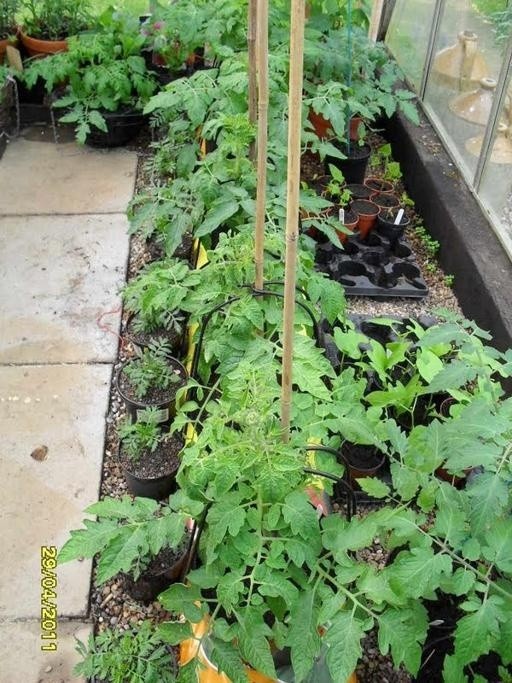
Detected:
[1,0,512,683]
